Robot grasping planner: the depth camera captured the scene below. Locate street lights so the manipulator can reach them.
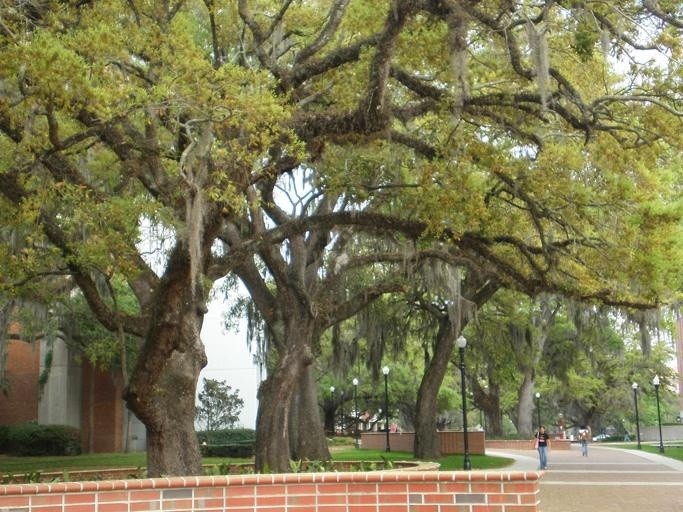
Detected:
[632,382,643,450]
[328,385,335,436]
[468,382,488,434]
[536,392,544,439]
[652,374,665,453]
[455,334,471,471]
[381,365,392,452]
[339,388,345,434]
[352,378,360,447]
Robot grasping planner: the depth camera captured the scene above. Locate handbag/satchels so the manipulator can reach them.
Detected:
[535,438,540,449]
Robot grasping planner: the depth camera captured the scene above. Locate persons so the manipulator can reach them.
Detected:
[533,424,550,469]
[579,430,590,458]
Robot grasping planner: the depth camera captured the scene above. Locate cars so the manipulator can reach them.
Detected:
[566,428,613,441]
